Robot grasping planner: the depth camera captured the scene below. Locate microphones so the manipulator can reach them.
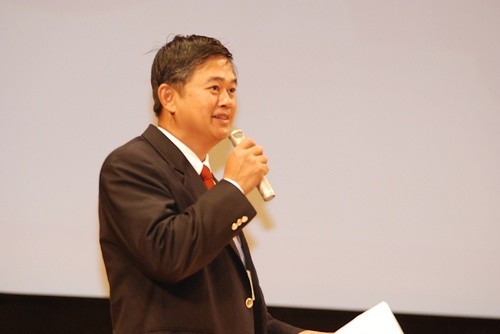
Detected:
[228,127,275,201]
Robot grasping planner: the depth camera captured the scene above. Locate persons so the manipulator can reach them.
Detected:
[97,33,333,334]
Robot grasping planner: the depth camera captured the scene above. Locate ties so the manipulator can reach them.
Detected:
[201,164,215,190]
